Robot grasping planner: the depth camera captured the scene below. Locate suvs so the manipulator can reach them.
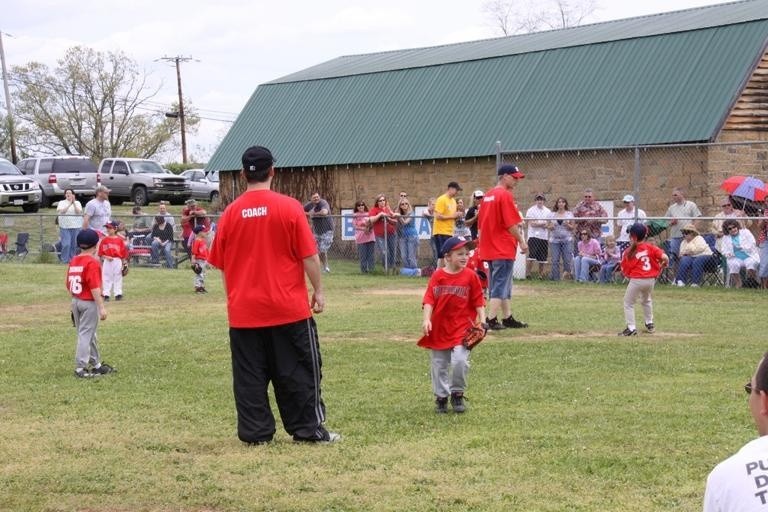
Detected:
[0,154,41,213]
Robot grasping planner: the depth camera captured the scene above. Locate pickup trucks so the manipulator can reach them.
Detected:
[102,154,190,206]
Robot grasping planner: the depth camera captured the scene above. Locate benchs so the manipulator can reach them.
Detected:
[139,237,190,269]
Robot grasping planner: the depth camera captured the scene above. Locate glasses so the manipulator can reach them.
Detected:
[727,225,736,231]
[721,203,731,207]
[744,383,760,394]
[358,194,408,207]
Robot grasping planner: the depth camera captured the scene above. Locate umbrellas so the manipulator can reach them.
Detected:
[719,175,768,211]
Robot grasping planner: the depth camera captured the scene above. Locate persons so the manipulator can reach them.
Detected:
[703,351,768,512]
[207,146,341,446]
[422,182,486,270]
[66,228,118,379]
[352,191,418,274]
[56,185,217,303]
[512,189,767,287]
[477,164,528,330]
[616,222,669,338]
[417,236,488,414]
[304,190,336,272]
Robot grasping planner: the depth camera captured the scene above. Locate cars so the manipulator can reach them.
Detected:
[176,166,222,201]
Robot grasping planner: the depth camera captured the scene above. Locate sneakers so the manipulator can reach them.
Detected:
[646,323,655,332]
[74,368,99,378]
[502,316,527,328]
[92,363,117,374]
[115,295,121,300]
[436,398,447,414]
[292,433,339,445]
[104,295,109,301]
[618,329,639,336]
[194,287,207,294]
[451,393,465,412]
[486,317,506,330]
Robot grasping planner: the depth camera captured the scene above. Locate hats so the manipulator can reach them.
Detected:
[474,190,484,196]
[194,225,209,234]
[242,146,276,172]
[440,236,477,253]
[448,181,463,190]
[626,224,647,237]
[77,229,103,249]
[680,224,697,232]
[622,195,634,202]
[103,219,117,228]
[95,185,111,194]
[497,165,525,178]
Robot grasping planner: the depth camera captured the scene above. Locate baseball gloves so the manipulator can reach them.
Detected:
[191,263,202,274]
[463,322,489,350]
[122,263,129,276]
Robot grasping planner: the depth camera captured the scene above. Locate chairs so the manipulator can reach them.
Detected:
[587,234,728,286]
[9,234,28,263]
[0,233,8,263]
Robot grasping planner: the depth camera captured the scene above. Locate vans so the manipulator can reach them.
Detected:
[15,155,100,205]
